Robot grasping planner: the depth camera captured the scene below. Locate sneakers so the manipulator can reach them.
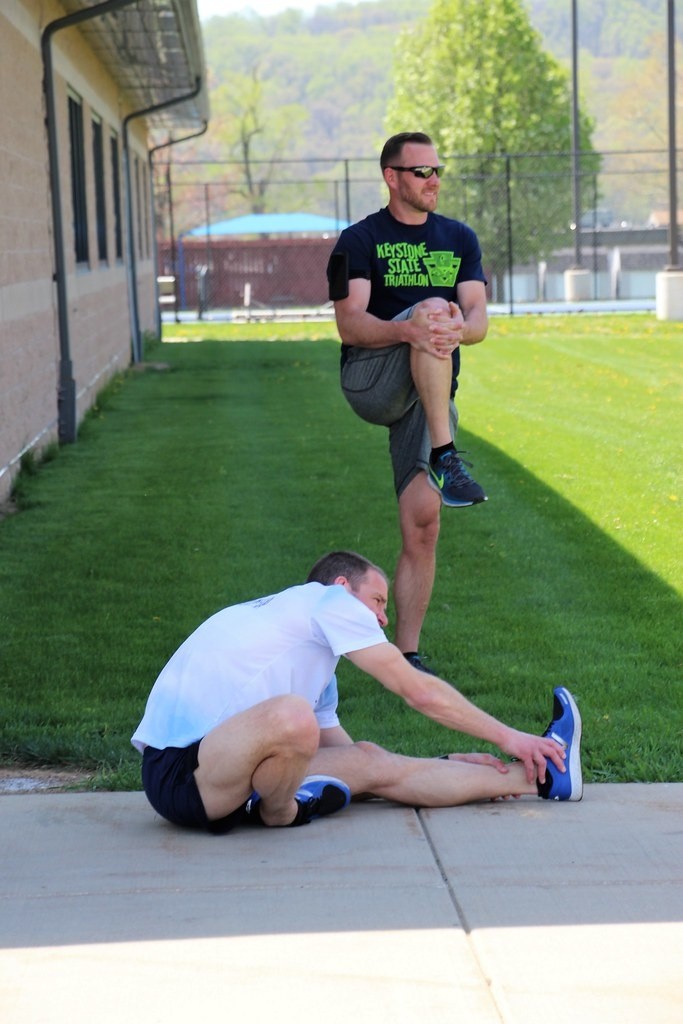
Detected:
[244,775,352,828]
[540,687,584,801]
[427,449,488,508]
[409,656,435,675]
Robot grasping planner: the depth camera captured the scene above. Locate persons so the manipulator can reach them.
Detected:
[324,132,492,672]
[130,547,587,838]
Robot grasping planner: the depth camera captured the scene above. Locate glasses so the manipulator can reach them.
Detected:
[390,166,445,179]
